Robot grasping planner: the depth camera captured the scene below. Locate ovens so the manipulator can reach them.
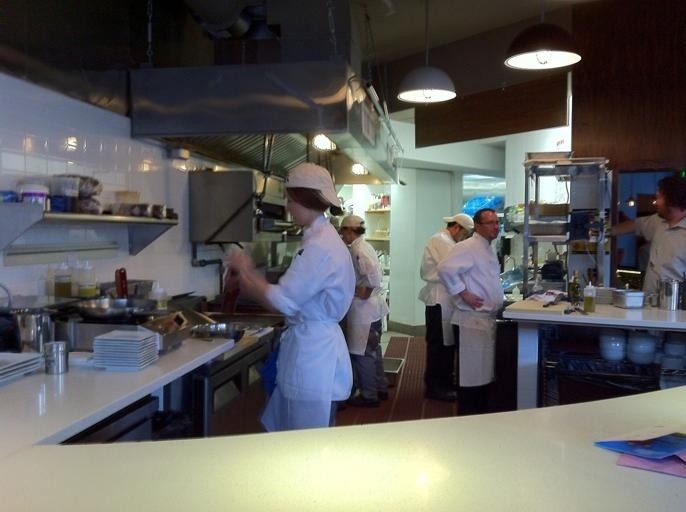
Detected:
[188,169,303,244]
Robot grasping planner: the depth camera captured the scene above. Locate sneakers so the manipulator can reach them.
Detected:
[378,391,389,400]
[425,389,458,402]
[346,393,381,407]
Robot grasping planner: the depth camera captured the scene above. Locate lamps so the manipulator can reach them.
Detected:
[504,0,581,74]
[396,2,456,103]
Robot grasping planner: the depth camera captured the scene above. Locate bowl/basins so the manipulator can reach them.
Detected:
[655,331,686,372]
[191,322,250,342]
[598,329,657,365]
[109,190,150,217]
[15,173,101,214]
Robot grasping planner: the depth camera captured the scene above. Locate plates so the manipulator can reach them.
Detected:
[0,352,42,385]
[596,287,615,304]
[93,329,160,371]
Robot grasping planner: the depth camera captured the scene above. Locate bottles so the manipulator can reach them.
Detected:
[570,270,596,313]
[368,192,389,209]
[512,285,521,299]
[532,274,544,295]
[52,258,97,298]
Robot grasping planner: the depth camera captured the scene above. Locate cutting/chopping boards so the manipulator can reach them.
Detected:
[506,299,571,316]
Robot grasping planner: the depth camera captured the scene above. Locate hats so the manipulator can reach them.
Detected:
[341,215,366,229]
[443,213,474,234]
[285,162,341,207]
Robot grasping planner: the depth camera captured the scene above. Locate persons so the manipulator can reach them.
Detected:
[334,212,391,408]
[218,161,356,436]
[605,177,686,307]
[417,209,472,403]
[433,207,502,418]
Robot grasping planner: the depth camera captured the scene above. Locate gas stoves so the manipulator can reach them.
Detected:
[74,308,186,327]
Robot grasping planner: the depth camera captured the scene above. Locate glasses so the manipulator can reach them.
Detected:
[481,221,499,224]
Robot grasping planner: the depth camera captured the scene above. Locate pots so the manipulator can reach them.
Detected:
[69,299,170,320]
[541,261,566,280]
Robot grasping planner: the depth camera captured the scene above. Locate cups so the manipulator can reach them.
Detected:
[155,292,167,310]
[43,341,69,375]
[649,288,659,307]
[374,248,391,267]
[152,205,177,220]
[17,313,50,352]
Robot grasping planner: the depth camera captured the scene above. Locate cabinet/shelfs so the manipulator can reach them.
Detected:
[363,207,391,243]
[523,157,607,299]
